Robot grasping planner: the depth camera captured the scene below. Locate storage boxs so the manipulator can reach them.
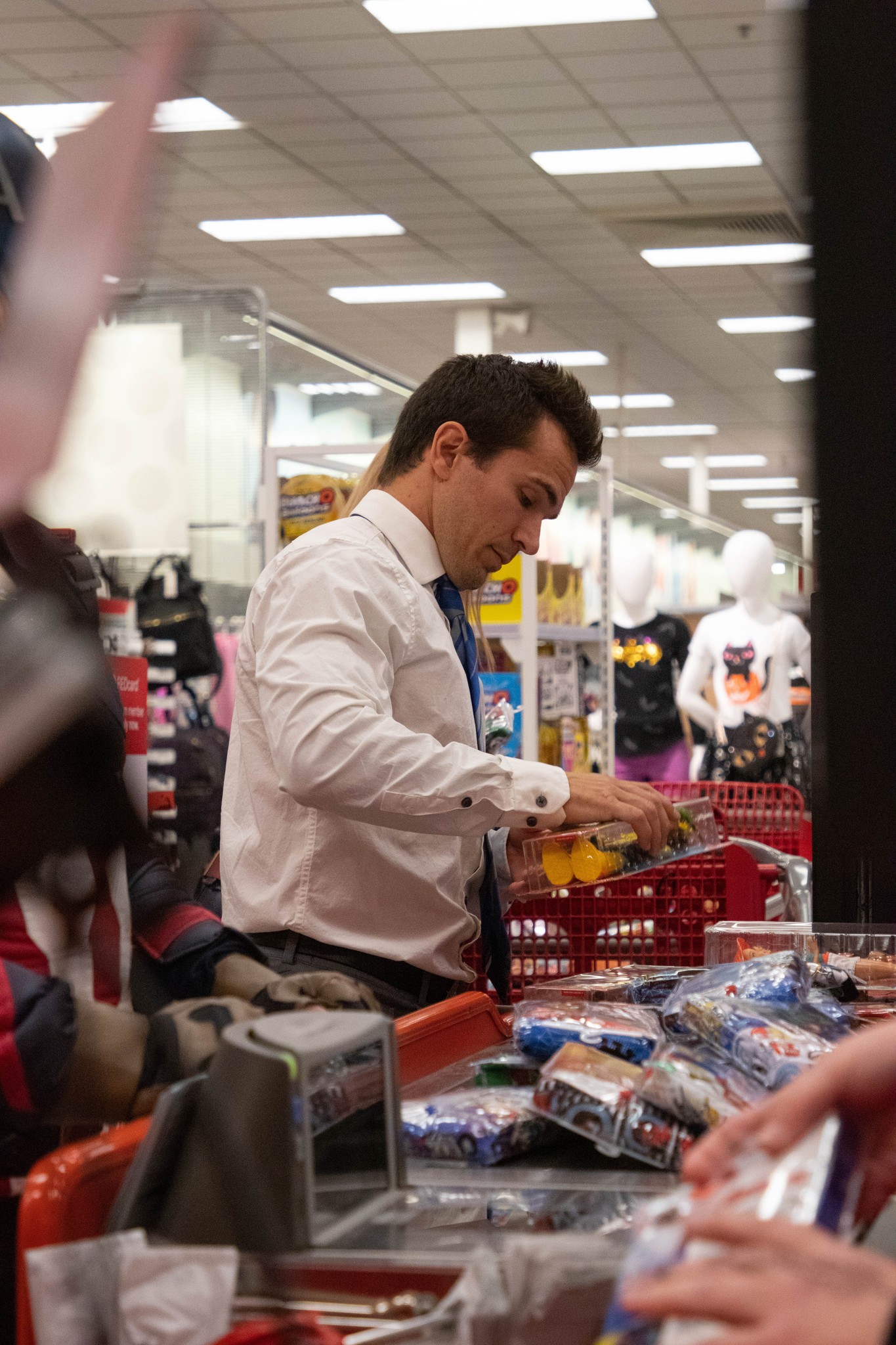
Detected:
[281,476,603,772]
[519,798,734,896]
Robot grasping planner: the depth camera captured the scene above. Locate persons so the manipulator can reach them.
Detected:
[587,535,708,800]
[674,528,811,809]
[0,108,381,1345]
[220,354,680,1021]
[614,1015,896,1345]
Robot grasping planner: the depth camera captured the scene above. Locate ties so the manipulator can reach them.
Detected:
[425,574,516,1011]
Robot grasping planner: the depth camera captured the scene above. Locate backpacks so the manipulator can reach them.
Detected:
[133,554,224,690]
[172,724,229,835]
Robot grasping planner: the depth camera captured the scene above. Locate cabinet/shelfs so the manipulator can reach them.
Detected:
[261,443,616,780]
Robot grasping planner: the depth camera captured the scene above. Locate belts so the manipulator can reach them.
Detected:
[250,929,459,1005]
